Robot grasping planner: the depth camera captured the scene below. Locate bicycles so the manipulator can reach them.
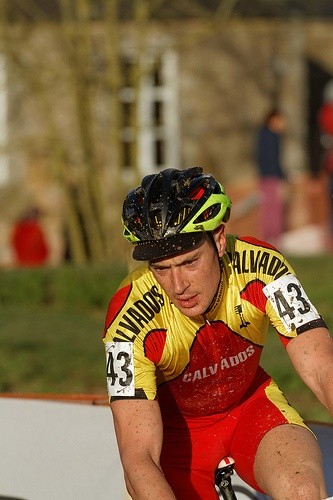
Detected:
[214,458,259,500]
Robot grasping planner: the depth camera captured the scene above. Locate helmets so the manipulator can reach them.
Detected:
[122,167,232,260]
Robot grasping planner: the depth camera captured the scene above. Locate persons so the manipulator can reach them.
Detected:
[254,87,333,254]
[103,166,333,500]
[10,206,50,268]
